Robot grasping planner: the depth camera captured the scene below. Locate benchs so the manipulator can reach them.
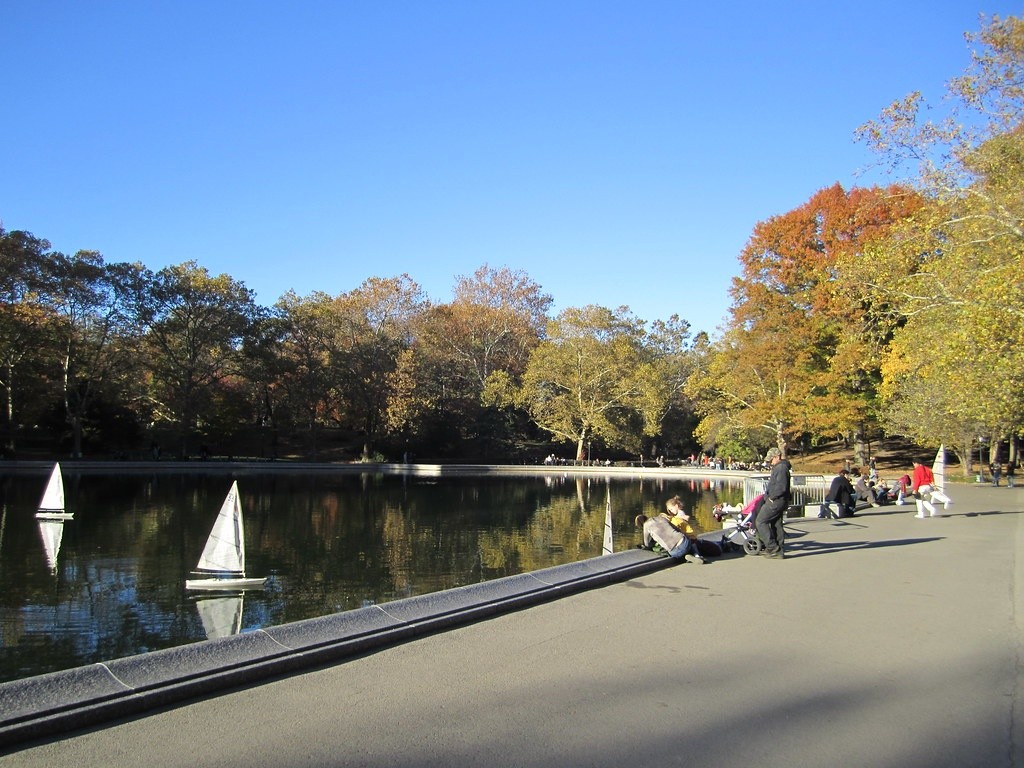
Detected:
[805,503,843,518]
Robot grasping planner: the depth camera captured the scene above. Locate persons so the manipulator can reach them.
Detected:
[826,458,937,519]
[991,459,1022,488]
[666,495,698,543]
[635,512,705,564]
[754,447,792,559]
[546,452,763,468]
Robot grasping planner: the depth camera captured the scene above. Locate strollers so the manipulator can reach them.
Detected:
[712,493,765,555]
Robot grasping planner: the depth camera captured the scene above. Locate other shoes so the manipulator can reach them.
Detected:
[848,510,856,517]
[685,554,704,564]
[871,503,880,508]
[930,508,935,516]
[696,556,707,563]
[914,515,919,518]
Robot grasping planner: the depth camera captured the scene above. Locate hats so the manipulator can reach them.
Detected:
[912,458,923,464]
[764,447,781,461]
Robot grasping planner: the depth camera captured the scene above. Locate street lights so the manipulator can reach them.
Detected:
[979,437,985,483]
[587,442,591,466]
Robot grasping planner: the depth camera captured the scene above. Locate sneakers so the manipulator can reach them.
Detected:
[761,545,779,555]
[767,552,784,559]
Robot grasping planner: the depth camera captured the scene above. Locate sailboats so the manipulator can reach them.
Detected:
[185,479,267,590]
[36,516,74,575]
[929,444,950,504]
[188,589,244,640]
[35,461,74,518]
